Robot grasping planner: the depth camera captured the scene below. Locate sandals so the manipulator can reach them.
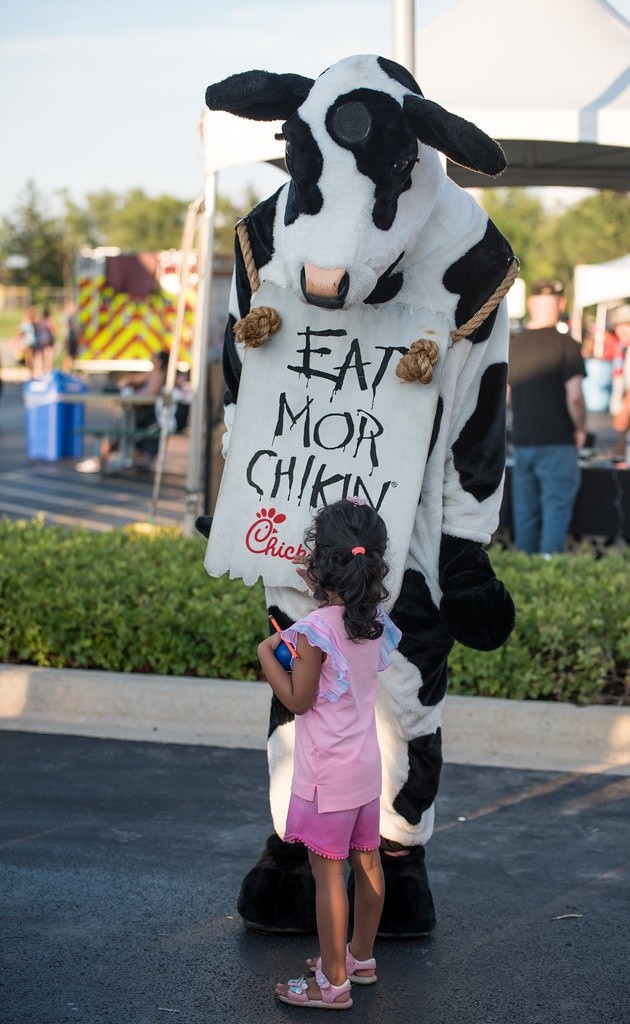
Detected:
[309,943,378,984]
[278,968,352,1008]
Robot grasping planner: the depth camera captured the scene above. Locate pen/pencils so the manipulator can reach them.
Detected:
[269,614,300,660]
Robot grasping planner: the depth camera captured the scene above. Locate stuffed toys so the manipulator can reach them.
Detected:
[197,47,519,938]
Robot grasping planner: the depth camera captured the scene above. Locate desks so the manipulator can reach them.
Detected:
[487,448,630,559]
[52,394,171,476]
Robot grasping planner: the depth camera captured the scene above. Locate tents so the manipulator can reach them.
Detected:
[569,253,630,359]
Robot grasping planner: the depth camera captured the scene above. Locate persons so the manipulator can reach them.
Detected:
[75,350,169,475]
[257,500,402,1008]
[506,276,588,556]
[18,306,56,378]
[525,278,630,469]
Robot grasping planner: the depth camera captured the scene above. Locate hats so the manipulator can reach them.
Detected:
[531,280,562,294]
